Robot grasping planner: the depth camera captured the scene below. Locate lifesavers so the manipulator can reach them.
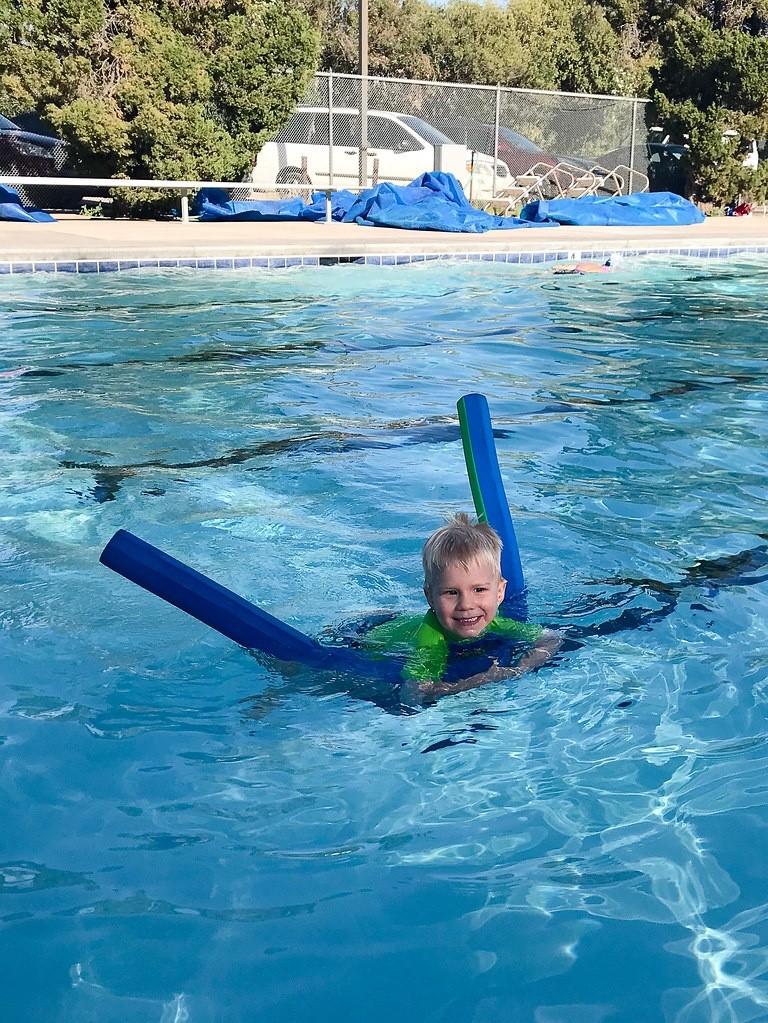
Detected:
[99,392,529,686]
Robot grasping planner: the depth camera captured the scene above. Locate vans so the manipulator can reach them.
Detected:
[248,101,516,206]
[650,126,759,173]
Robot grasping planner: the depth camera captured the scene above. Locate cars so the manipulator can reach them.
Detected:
[426,116,562,185]
[593,141,690,196]
[0,115,79,176]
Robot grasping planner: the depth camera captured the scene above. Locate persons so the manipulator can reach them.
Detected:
[420,512,508,648]
